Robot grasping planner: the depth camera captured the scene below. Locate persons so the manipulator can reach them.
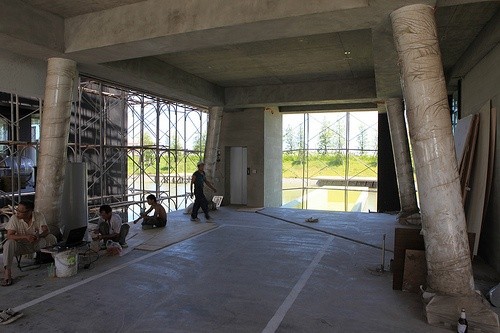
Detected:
[190,162,218,221]
[1,201,60,285]
[93,205,130,248]
[133,194,167,229]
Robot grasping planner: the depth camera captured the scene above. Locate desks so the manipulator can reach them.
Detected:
[393,227,476,290]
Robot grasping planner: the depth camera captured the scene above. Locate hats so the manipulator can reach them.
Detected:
[197,161,205,166]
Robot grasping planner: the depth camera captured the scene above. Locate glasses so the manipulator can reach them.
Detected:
[15,209,29,215]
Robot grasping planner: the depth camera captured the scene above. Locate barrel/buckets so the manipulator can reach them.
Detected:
[55,250,78,278]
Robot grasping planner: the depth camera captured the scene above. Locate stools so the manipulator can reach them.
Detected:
[17,252,42,272]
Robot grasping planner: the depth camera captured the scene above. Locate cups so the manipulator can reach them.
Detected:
[141,208,145,214]
[47,266,56,281]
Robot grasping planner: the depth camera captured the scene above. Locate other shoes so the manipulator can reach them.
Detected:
[143,224,153,230]
[101,243,107,249]
[0,308,24,325]
[191,217,200,221]
[205,214,211,220]
[120,241,128,247]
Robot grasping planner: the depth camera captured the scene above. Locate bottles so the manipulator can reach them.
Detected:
[89,229,123,257]
[457,308,468,333]
